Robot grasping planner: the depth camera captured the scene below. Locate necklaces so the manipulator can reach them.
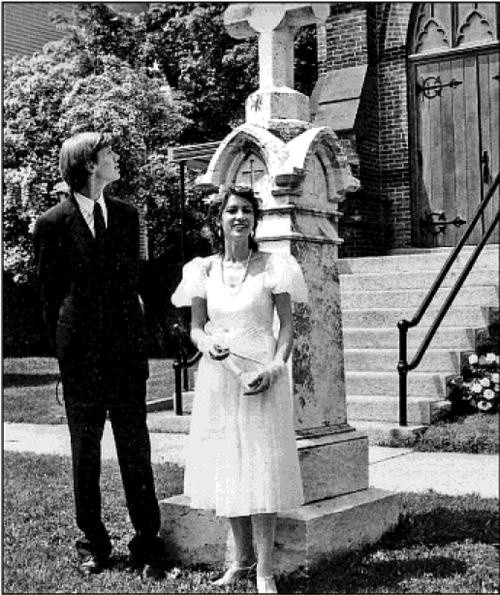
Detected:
[220,248,253,287]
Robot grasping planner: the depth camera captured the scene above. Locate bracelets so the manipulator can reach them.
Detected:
[197,336,215,359]
[267,359,286,387]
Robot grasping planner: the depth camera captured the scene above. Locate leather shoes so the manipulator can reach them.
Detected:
[79,555,104,578]
[140,563,167,584]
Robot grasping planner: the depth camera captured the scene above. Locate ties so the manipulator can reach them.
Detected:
[94,202,106,239]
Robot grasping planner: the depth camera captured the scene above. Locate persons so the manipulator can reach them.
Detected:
[166,183,309,594]
[18,129,178,583]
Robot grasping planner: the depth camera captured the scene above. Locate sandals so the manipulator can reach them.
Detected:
[212,563,257,587]
[255,575,277,594]
